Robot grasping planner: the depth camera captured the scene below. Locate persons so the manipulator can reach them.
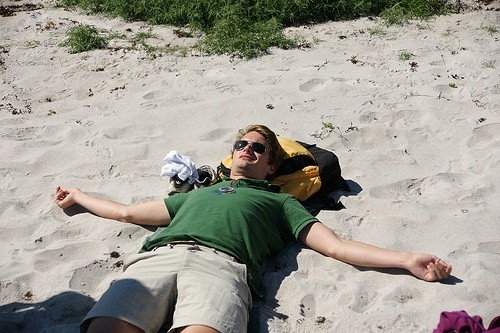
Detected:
[55,125,452,333]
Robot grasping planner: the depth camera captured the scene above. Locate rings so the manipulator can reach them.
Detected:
[437,259,441,262]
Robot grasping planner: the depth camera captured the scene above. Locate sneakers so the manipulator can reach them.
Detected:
[168,174,193,196]
[193,164,219,189]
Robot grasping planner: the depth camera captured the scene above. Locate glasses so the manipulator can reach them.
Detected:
[234,139,266,154]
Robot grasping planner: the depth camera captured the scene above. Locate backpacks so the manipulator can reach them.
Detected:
[217,134,350,210]
